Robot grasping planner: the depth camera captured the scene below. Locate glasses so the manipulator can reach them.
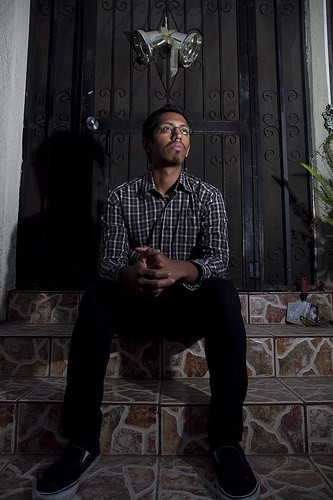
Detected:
[300,315,325,327]
[153,126,193,135]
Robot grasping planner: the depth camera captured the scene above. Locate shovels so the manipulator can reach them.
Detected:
[286,276,318,326]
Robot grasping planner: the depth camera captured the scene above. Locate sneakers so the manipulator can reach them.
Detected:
[33,444,100,500]
[210,444,260,500]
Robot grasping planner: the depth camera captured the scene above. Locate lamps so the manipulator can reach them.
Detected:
[122,17,203,68]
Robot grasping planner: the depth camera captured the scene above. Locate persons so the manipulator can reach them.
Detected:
[32,106,261,500]
